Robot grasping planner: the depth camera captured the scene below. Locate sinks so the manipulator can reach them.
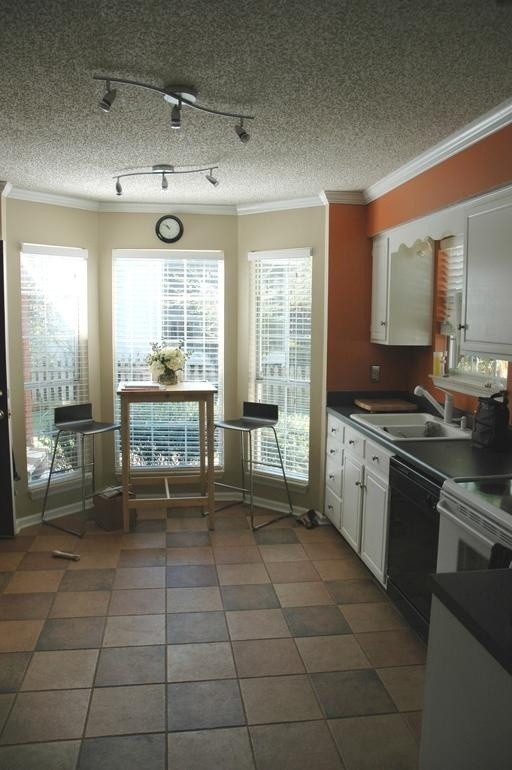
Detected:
[370,426,473,443]
[349,412,445,428]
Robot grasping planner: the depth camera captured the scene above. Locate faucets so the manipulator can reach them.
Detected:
[414,385,455,423]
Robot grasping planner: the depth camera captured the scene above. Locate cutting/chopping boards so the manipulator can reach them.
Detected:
[353,397,419,412]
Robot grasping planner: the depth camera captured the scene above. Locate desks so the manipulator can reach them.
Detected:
[116,380,218,532]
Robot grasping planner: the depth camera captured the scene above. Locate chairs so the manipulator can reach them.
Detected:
[42,403,131,539]
[200,401,295,529]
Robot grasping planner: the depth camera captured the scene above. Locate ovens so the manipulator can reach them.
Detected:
[433,488,512,574]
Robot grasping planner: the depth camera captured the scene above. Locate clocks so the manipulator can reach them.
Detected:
[154,215,184,243]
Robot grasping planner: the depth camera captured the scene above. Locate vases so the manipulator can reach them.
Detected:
[156,372,179,384]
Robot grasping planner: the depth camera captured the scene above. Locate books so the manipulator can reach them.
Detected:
[121,382,159,391]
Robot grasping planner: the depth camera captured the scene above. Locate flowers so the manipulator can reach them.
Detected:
[144,338,195,376]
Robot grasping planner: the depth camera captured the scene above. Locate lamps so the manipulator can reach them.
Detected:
[112,165,219,196]
[92,75,254,145]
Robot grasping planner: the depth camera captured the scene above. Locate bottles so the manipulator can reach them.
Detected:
[433,350,450,378]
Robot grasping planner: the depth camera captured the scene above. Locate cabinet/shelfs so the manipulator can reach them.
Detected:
[370,184,512,362]
[323,406,395,593]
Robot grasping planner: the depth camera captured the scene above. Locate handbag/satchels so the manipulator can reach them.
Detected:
[472,397,509,449]
